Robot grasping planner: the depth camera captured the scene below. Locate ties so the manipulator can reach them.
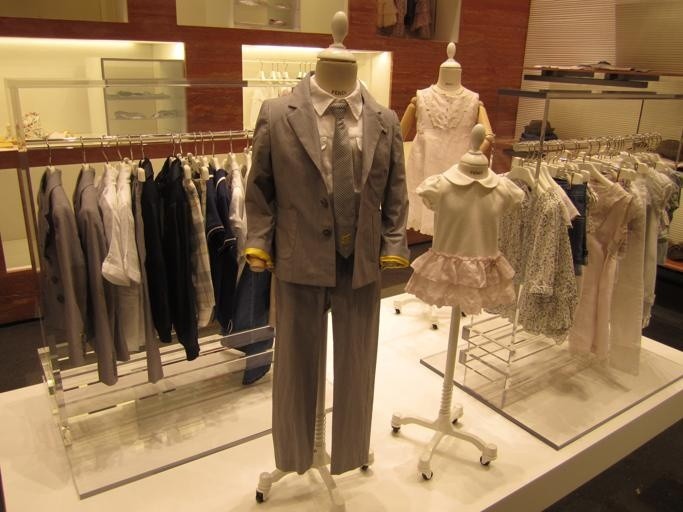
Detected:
[330,102,355,258]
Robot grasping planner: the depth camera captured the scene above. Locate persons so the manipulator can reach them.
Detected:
[242,46,411,476]
[405,150,525,314]
[398,58,494,238]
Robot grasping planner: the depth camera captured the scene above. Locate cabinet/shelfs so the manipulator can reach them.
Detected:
[86,58,185,135]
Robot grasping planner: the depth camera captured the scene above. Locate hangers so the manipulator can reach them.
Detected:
[46,129,252,181]
[259,61,316,79]
[501,133,663,194]
